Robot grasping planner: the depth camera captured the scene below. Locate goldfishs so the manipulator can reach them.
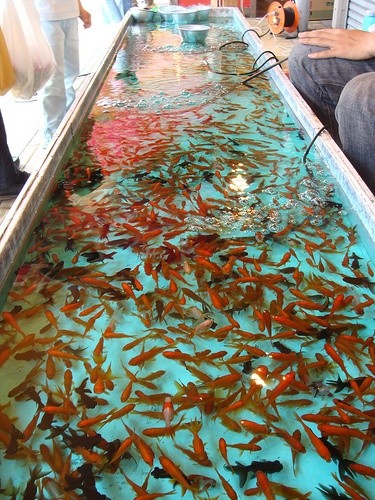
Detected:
[224,458,283,488]
[0,16,375,500]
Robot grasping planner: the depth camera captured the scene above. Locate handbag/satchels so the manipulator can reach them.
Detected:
[0,0,57,100]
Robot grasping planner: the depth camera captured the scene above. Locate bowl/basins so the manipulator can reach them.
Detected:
[178,24,210,44]
[129,5,210,25]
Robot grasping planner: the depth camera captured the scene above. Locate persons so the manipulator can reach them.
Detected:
[287,27,375,194]
[0,107,31,201]
[34,0,91,151]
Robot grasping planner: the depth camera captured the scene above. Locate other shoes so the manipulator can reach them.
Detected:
[0,167,31,200]
[13,157,20,167]
[43,141,49,149]
